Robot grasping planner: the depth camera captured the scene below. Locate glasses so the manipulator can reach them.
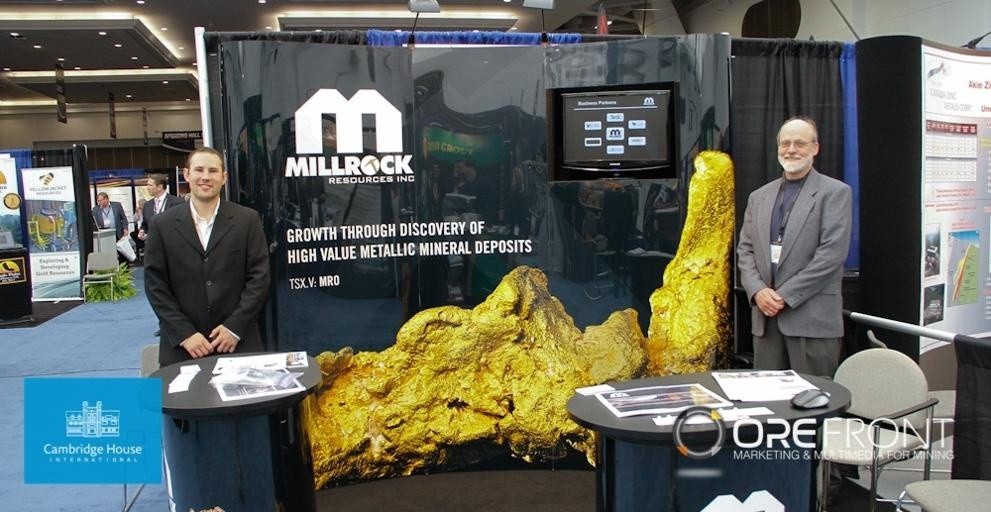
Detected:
[777,139,815,148]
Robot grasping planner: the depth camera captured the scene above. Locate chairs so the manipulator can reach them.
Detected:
[82,253,120,304]
[818,348,938,511]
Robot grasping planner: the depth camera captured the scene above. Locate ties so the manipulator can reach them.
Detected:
[152,198,160,214]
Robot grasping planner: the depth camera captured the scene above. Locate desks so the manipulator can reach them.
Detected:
[566,369,853,511]
[137,351,322,510]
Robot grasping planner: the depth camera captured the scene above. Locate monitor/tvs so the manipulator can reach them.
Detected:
[545,79,684,181]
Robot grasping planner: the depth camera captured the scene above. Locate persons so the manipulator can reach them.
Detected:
[137,173,184,241]
[92,192,129,274]
[736,116,852,504]
[144,146,271,369]
[130,199,147,247]
